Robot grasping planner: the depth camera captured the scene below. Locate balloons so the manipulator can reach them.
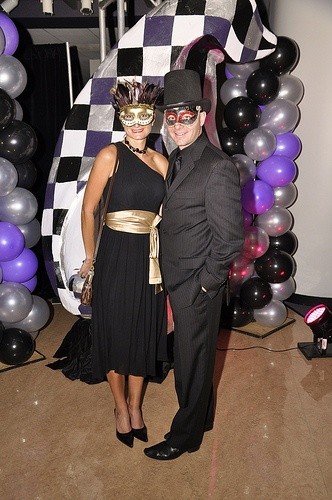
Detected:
[0,13,50,364]
[228,154,296,328]
[221,35,304,162]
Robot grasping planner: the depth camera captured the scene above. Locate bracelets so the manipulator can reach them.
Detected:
[82,258,96,263]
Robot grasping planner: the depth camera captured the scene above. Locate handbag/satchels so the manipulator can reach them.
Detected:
[80,267,95,305]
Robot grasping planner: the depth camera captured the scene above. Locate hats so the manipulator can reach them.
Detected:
[154,69,211,115]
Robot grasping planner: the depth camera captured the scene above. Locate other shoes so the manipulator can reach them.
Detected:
[116,429,134,448]
[133,427,147,442]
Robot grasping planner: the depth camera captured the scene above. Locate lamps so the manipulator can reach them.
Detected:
[40,0,53,17]
[0,0,18,15]
[79,0,93,16]
[297,303,332,360]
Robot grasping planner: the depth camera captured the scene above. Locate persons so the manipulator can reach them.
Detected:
[45,77,168,448]
[144,69,245,462]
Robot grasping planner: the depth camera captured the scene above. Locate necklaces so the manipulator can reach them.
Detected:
[124,137,148,154]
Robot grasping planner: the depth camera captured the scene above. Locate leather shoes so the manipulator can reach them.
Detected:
[145,438,201,460]
[164,422,213,440]
